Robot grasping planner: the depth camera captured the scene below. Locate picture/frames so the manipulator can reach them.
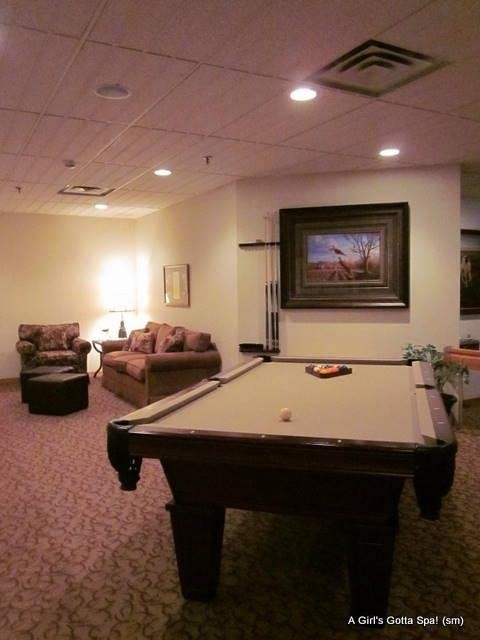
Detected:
[279,201,410,309]
[460,229,480,314]
[163,264,190,309]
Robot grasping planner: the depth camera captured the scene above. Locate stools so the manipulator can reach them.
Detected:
[20,364,89,416]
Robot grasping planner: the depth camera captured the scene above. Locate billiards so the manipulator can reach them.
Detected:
[306,364,349,378]
[279,407,292,421]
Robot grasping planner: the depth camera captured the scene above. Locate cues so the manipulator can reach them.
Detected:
[265,211,279,352]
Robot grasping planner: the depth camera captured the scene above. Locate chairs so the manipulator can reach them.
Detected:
[18,323,92,406]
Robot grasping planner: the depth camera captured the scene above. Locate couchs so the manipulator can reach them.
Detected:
[103,322,221,411]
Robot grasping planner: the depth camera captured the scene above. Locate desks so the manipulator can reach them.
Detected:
[106,355,457,625]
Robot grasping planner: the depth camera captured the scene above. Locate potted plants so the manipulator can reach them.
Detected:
[404,343,470,418]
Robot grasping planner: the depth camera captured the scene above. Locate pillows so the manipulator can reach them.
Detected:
[123,328,185,357]
